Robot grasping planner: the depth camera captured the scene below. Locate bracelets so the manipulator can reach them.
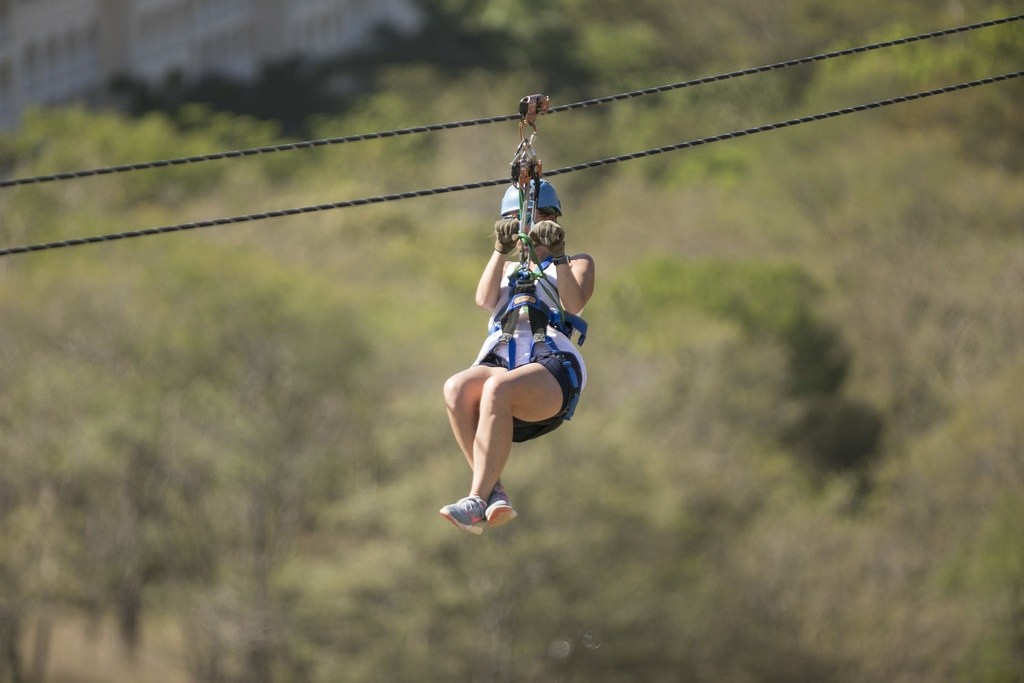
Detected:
[552,255,570,265]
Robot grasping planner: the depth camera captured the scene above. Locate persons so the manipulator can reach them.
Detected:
[439,177,595,536]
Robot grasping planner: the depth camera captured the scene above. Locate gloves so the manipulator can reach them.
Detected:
[494,218,522,255]
[529,219,566,258]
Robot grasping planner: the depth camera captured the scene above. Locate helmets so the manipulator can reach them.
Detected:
[500,178,562,218]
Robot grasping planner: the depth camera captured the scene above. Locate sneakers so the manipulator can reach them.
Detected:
[484,481,517,528]
[439,493,487,534]
